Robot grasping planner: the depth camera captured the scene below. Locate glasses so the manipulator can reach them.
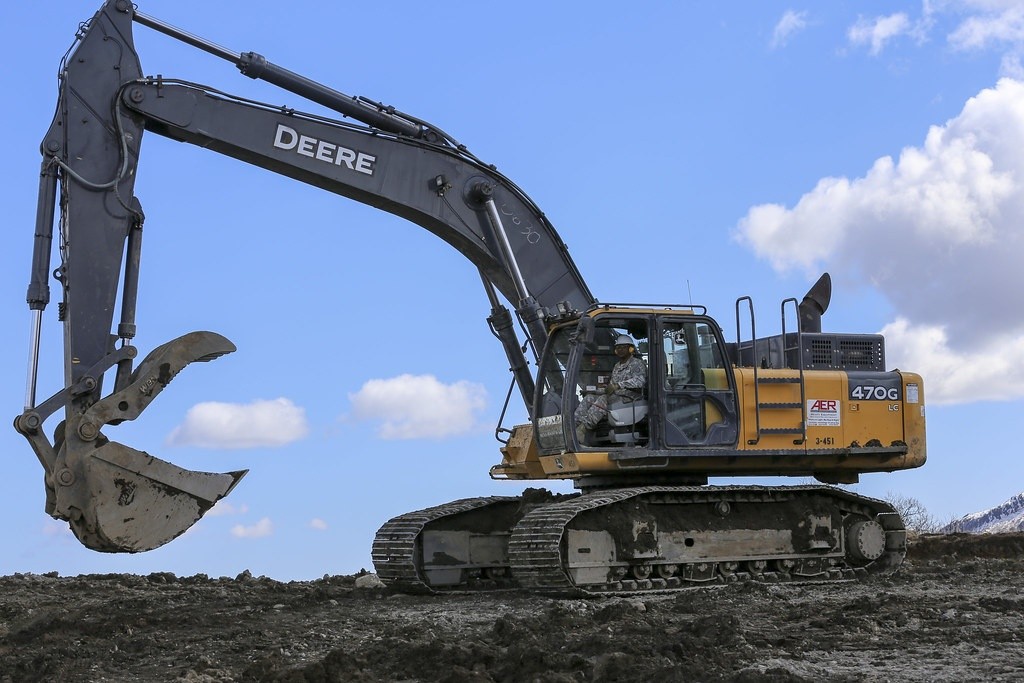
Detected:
[616,346,628,349]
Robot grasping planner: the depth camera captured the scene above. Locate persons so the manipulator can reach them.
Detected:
[573,335,648,445]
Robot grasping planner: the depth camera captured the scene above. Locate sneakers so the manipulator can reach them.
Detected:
[575,421,587,443]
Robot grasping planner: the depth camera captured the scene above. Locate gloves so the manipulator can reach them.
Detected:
[605,383,620,395]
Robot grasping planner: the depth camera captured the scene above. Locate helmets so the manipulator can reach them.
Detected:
[614,335,634,345]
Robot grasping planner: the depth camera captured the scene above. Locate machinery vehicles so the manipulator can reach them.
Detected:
[11,0,928,596]
